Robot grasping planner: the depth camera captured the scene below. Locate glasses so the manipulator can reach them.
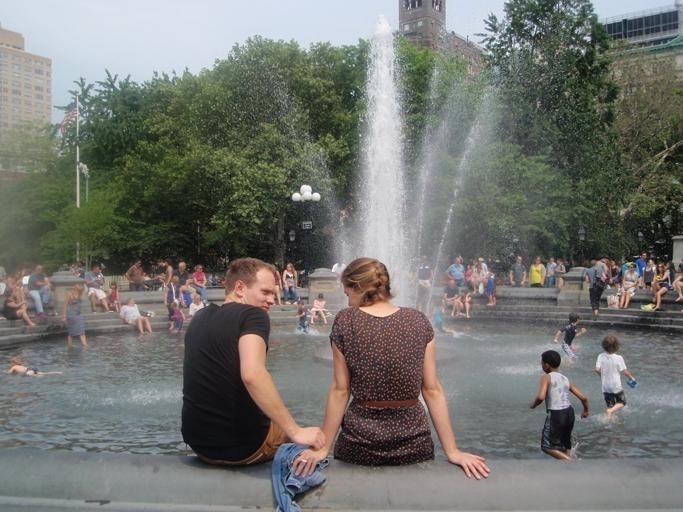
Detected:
[658,265,664,268]
[629,266,635,269]
[196,267,203,269]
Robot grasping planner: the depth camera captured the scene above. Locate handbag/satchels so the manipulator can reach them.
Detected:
[594,269,607,290]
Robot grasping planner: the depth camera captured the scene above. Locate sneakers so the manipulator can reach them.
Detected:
[652,305,662,311]
[594,311,600,322]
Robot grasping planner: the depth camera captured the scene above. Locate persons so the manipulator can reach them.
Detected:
[273,270,283,305]
[652,261,675,311]
[440,279,460,318]
[553,312,587,367]
[48,282,59,315]
[443,256,466,288]
[636,252,647,291]
[144,272,155,281]
[484,270,497,306]
[155,258,174,306]
[584,260,606,321]
[190,264,209,306]
[124,258,150,291]
[332,257,347,294]
[621,256,640,278]
[414,255,434,318]
[290,258,491,480]
[619,264,638,309]
[108,281,121,313]
[428,298,446,335]
[210,274,218,287]
[3,288,39,326]
[553,258,566,287]
[26,265,52,321]
[607,260,620,289]
[470,263,489,294]
[0,265,29,303]
[528,350,589,460]
[173,302,186,333]
[310,293,327,324]
[155,272,167,288]
[167,275,179,325]
[120,296,153,334]
[71,264,80,279]
[529,256,546,287]
[595,336,637,418]
[295,300,310,335]
[546,257,557,287]
[464,265,473,290]
[173,262,189,298]
[597,257,609,276]
[84,262,115,313]
[455,288,472,319]
[653,259,666,279]
[645,258,655,292]
[187,293,205,317]
[5,355,63,379]
[62,283,87,347]
[282,262,299,304]
[180,257,328,467]
[176,278,197,306]
[77,261,86,280]
[672,263,683,302]
[509,256,526,287]
[300,263,312,289]
[477,257,489,276]
[173,290,182,309]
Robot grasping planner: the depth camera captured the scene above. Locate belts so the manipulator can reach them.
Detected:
[351,398,423,408]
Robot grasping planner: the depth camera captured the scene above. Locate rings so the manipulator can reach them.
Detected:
[300,459,308,462]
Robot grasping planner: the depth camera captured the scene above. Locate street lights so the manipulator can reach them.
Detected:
[78,162,89,204]
[291,184,322,270]
